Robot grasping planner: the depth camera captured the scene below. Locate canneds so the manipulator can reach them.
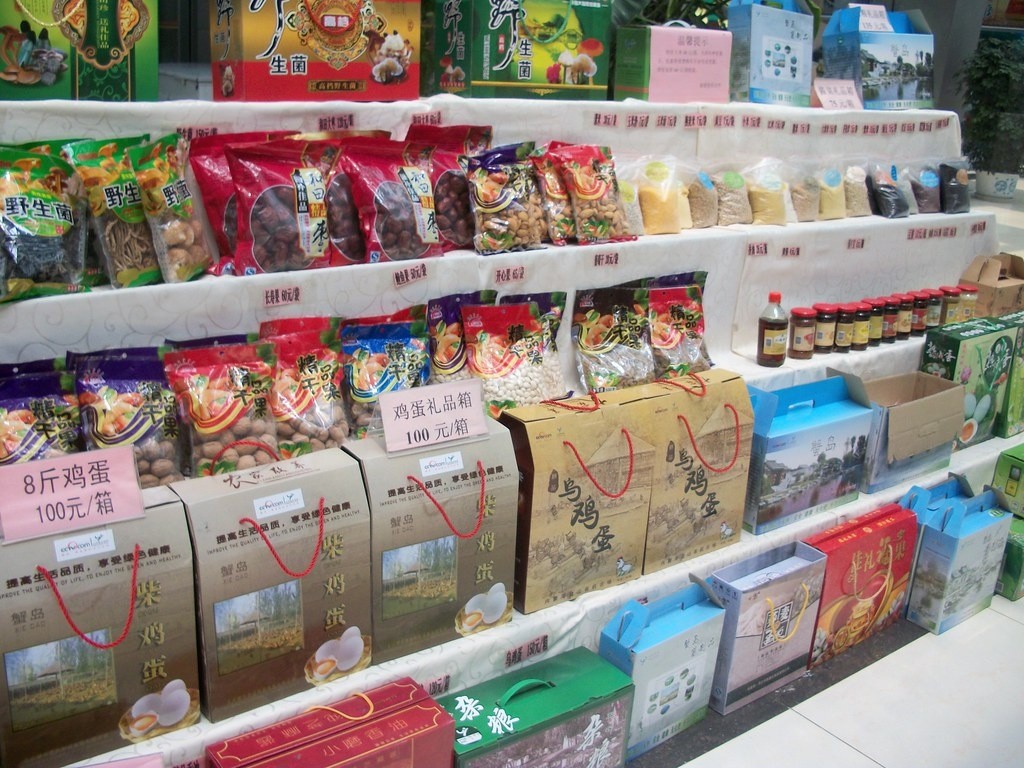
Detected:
[787,284,980,359]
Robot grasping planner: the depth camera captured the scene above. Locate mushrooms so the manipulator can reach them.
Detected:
[1,170,209,296]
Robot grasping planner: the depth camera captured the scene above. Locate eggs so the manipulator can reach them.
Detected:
[462,582,507,630]
[958,392,991,445]
[128,679,192,737]
[313,626,365,678]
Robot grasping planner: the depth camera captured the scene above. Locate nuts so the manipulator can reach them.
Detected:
[476,145,627,256]
[1,306,713,500]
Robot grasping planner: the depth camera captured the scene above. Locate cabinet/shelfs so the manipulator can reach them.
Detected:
[1,0,1024,768]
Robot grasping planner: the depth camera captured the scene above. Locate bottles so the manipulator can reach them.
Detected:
[755,291,789,368]
